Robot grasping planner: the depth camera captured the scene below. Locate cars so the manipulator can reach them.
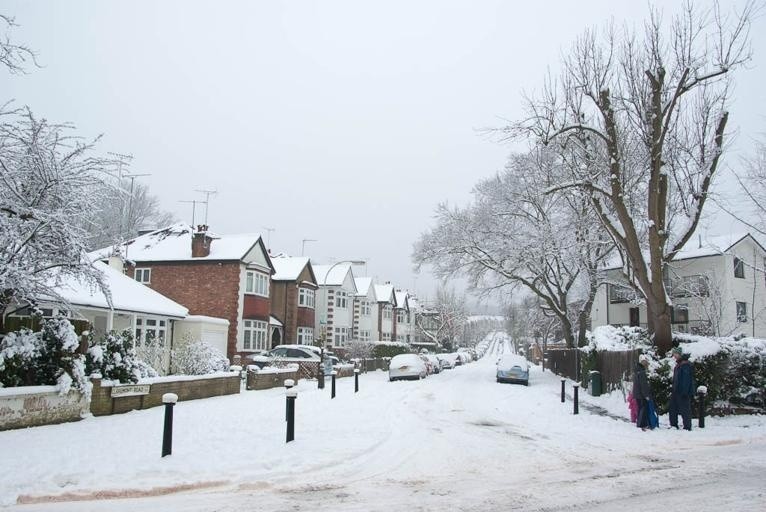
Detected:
[496,354,531,386]
[389,340,488,381]
[244,345,338,373]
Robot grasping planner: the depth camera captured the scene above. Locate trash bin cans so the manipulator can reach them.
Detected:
[588,371,600,396]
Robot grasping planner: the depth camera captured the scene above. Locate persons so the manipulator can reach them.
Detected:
[632,354,660,432]
[666,347,686,395]
[668,354,692,432]
[626,388,639,422]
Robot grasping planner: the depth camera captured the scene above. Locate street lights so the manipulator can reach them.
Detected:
[322,260,366,323]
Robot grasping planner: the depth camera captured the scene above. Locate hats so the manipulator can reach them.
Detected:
[672,347,682,354]
[638,354,646,363]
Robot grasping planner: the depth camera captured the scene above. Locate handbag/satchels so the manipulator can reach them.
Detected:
[645,400,658,430]
